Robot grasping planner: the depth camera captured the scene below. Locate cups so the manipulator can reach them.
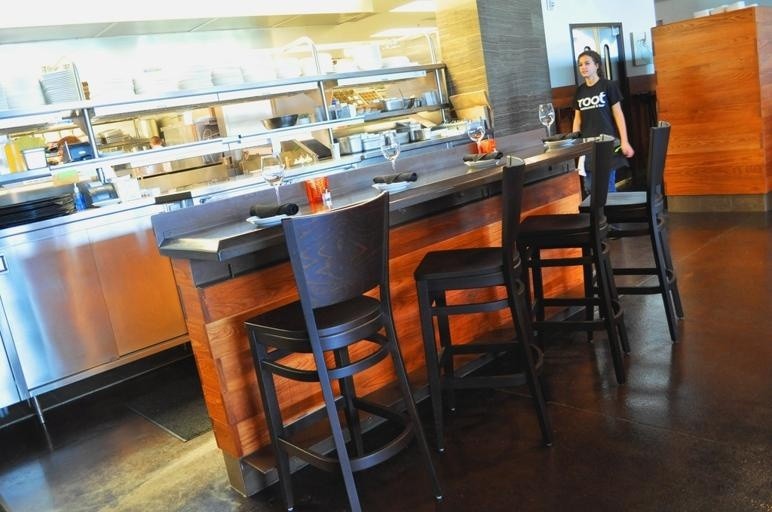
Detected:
[467,119,486,158]
[260,153,286,206]
[304,176,330,205]
[476,138,496,155]
[538,103,556,137]
[379,129,401,176]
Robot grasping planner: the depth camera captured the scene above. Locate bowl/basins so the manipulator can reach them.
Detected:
[260,114,299,130]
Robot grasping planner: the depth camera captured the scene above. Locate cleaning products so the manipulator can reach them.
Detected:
[72,182,86,213]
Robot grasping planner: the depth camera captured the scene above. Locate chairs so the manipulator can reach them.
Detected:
[579,120,685,345]
[517,134,632,384]
[243,190,446,512]
[413,155,553,452]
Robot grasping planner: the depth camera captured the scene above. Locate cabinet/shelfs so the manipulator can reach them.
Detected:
[651,6,772,211]
[0,62,493,452]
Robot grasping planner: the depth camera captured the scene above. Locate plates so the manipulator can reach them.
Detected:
[240,48,279,84]
[383,55,411,69]
[301,53,336,76]
[345,42,387,72]
[3,76,46,110]
[274,56,304,80]
[38,65,85,105]
[543,138,577,147]
[132,74,157,95]
[334,58,360,74]
[210,66,245,87]
[176,61,216,91]
[370,182,415,192]
[144,66,181,95]
[90,197,123,208]
[245,213,293,227]
[463,158,500,167]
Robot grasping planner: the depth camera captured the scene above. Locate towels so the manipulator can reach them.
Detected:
[541,131,581,142]
[462,150,504,160]
[373,172,419,184]
[249,202,299,218]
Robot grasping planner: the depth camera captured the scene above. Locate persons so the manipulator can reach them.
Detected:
[571,50,635,193]
